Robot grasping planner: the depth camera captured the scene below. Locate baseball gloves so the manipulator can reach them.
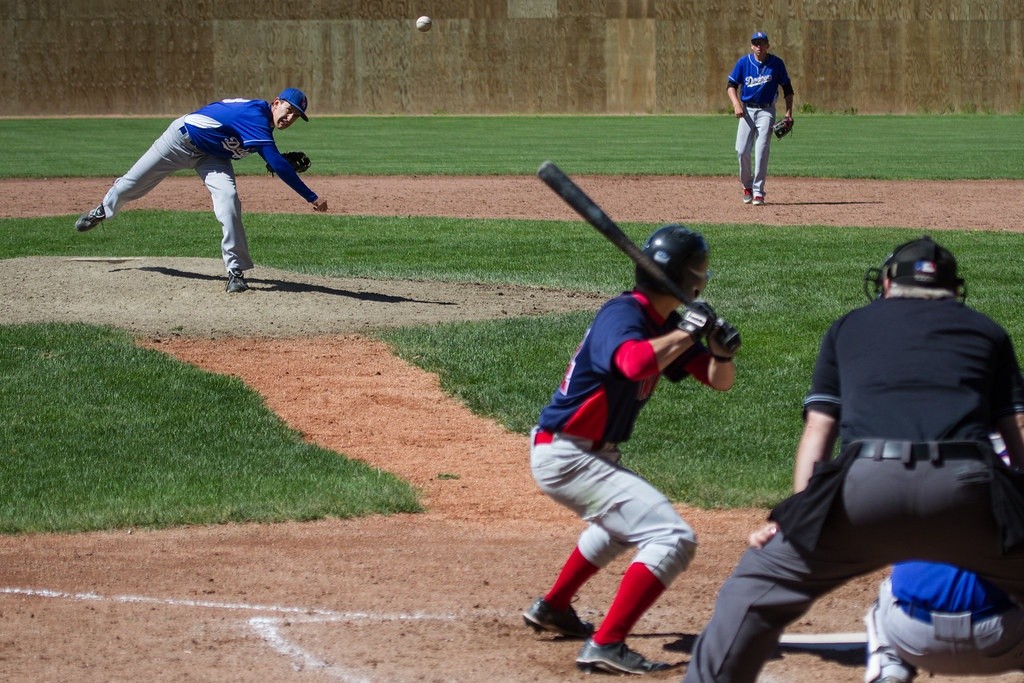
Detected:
[773,116,793,137]
[265,150,313,174]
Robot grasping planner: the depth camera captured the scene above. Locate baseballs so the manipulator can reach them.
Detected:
[415,15,432,31]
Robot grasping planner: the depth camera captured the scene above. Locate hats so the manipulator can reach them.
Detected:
[278,87,309,122]
[750,32,768,41]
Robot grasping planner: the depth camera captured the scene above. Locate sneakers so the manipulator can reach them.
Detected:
[752,196,764,205]
[575,640,670,674]
[74,204,106,233]
[743,188,752,203]
[522,595,595,641]
[225,268,248,294]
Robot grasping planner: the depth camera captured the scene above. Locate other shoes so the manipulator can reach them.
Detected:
[872,666,908,683]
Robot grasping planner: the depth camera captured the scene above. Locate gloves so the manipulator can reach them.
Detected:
[677,306,717,345]
[707,318,741,362]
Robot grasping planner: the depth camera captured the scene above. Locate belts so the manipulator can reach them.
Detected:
[534,432,604,452]
[859,442,985,460]
[180,126,196,148]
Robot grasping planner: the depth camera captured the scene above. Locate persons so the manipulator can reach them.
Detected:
[75,89,328,294]
[523,224,743,676]
[727,31,794,206]
[681,236,1024,683]
[863,561,1024,683]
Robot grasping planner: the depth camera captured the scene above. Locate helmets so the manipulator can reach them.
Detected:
[864,234,967,304]
[639,225,709,302]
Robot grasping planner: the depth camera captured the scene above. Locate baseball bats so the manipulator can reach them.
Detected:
[535,158,725,330]
